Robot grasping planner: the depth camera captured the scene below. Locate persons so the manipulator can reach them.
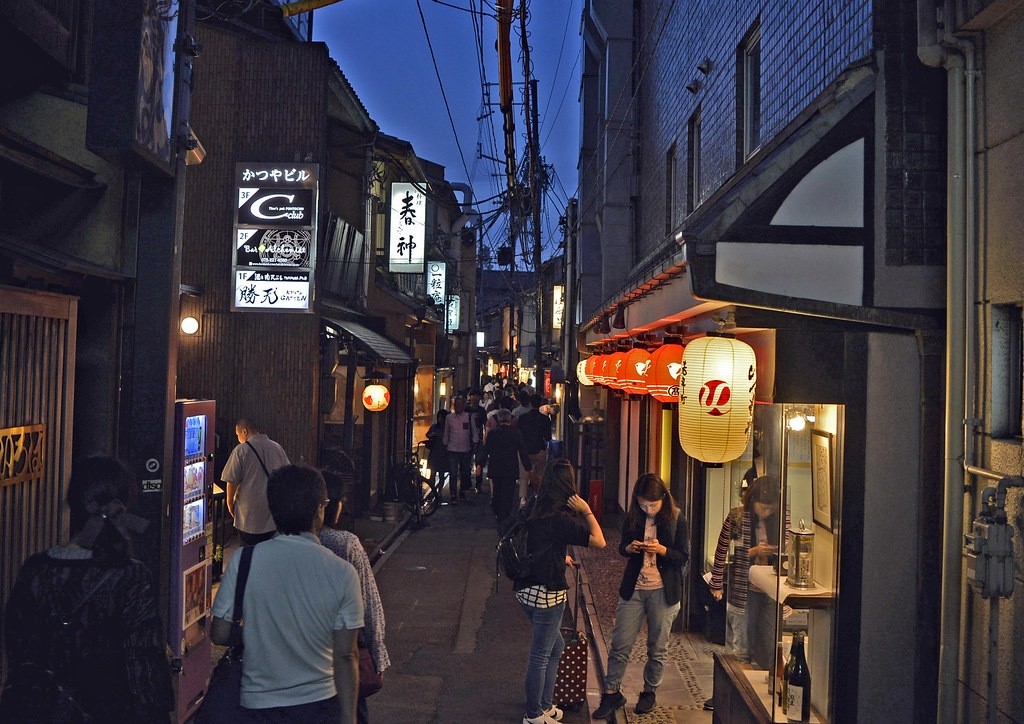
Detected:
[703,468,758,711]
[316,471,391,724]
[5,456,174,724]
[582,408,602,422]
[425,372,560,548]
[184,465,204,493]
[219,418,290,544]
[210,464,364,724]
[746,477,780,710]
[512,459,607,724]
[592,473,690,718]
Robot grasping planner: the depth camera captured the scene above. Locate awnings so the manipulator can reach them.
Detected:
[321,315,415,364]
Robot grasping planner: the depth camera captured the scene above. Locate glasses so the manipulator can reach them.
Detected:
[323,498,330,507]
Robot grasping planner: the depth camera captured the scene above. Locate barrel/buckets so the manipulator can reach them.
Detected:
[384,501,400,521]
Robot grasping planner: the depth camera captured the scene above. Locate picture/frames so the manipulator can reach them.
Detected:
[809,428,835,534]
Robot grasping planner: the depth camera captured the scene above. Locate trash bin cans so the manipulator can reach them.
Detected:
[704,559,732,645]
[327,474,355,533]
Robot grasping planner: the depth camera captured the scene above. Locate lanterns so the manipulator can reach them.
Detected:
[585,344,651,400]
[679,331,756,468]
[577,358,594,386]
[362,385,389,411]
[644,338,685,410]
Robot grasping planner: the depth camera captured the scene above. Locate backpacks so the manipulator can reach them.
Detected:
[496,509,555,580]
[1,567,119,724]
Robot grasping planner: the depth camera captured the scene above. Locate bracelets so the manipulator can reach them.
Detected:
[584,513,594,519]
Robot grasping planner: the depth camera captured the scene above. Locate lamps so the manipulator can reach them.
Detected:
[612,302,626,329]
[786,404,807,432]
[600,312,611,334]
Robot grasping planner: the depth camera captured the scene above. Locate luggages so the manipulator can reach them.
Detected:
[552,559,589,712]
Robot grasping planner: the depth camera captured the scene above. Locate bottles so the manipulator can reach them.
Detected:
[782,629,811,724]
[768,642,784,695]
[183,505,199,529]
[185,417,200,454]
[184,464,204,491]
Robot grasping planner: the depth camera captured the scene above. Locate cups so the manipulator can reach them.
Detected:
[773,553,789,576]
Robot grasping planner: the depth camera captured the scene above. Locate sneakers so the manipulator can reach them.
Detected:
[637,692,656,713]
[523,712,562,724]
[593,692,626,719]
[547,705,564,721]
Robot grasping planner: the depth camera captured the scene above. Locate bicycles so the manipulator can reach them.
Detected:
[393,441,440,529]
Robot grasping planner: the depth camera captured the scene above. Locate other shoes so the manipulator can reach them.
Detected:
[439,492,443,498]
[704,699,713,710]
[518,499,526,514]
[460,492,465,501]
[430,491,434,496]
[475,486,483,495]
[451,495,457,504]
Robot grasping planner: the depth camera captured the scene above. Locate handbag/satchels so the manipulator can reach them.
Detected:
[426,423,436,449]
[356,646,383,699]
[194,649,241,723]
